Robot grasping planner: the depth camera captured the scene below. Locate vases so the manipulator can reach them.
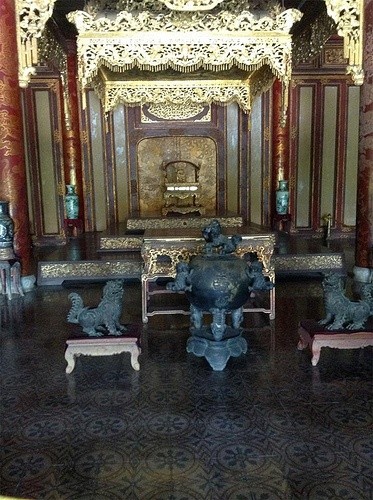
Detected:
[64,185,81,220]
[0,200,16,261]
[275,180,289,215]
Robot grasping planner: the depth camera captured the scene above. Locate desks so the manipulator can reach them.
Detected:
[271,213,291,241]
[296,318,373,367]
[64,323,142,374]
[139,225,278,325]
[63,218,83,245]
[0,260,25,300]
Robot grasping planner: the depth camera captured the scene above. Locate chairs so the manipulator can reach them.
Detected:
[158,158,208,216]
